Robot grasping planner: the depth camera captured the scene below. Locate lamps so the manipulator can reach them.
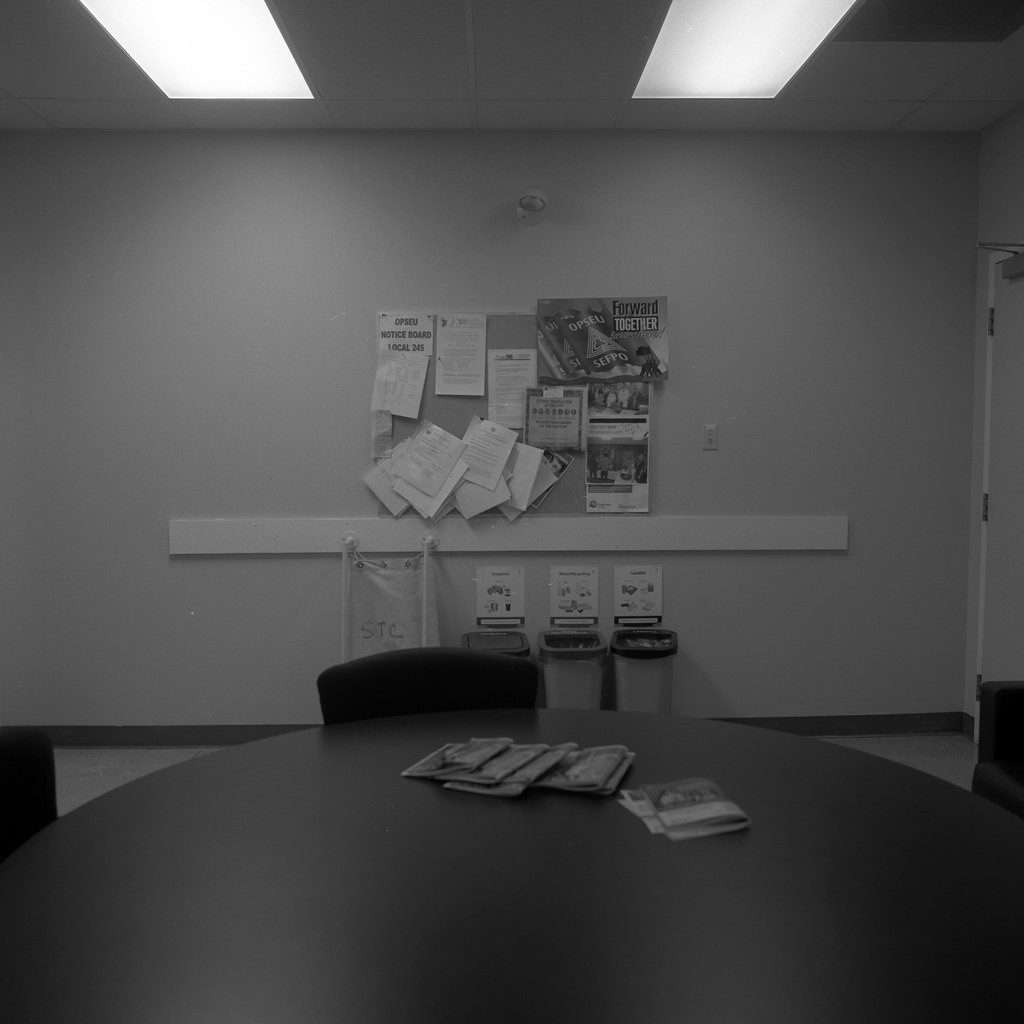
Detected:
[517,186,547,224]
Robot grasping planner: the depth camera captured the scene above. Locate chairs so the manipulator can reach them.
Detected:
[317,646,546,724]
[0,727,58,867]
[971,681,1024,822]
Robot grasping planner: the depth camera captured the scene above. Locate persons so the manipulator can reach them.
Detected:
[545,381,648,483]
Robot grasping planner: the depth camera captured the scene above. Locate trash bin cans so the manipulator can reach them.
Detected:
[609,628,678,714]
[536,628,608,711]
[460,631,531,657]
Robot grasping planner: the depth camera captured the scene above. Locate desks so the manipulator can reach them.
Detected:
[0,709,1024,1024]
